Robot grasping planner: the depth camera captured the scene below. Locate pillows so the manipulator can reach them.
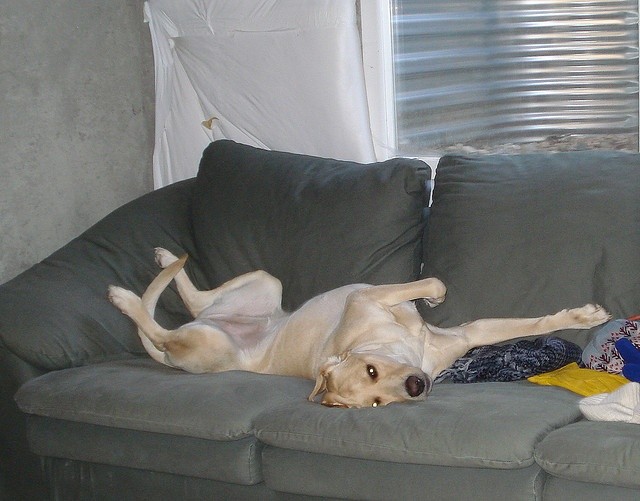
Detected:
[190,141,429,311]
[412,150,638,328]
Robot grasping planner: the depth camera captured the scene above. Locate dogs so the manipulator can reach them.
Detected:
[105,244,610,409]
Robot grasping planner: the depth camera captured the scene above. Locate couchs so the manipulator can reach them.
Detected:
[2,138,639,500]
